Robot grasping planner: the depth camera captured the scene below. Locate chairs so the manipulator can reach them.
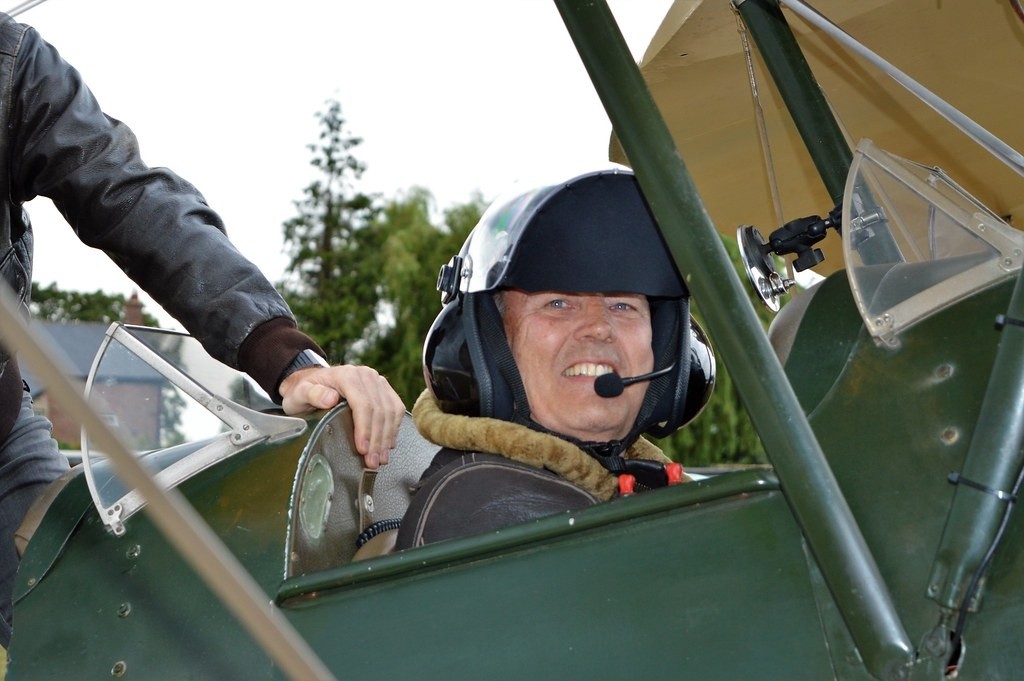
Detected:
[285,404,444,588]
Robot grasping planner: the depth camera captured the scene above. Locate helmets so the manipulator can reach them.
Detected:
[435,161,692,437]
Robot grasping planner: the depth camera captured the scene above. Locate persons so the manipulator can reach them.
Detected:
[0,7,408,649]
[392,161,691,555]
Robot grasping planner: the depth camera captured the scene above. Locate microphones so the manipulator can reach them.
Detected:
[594,362,678,398]
